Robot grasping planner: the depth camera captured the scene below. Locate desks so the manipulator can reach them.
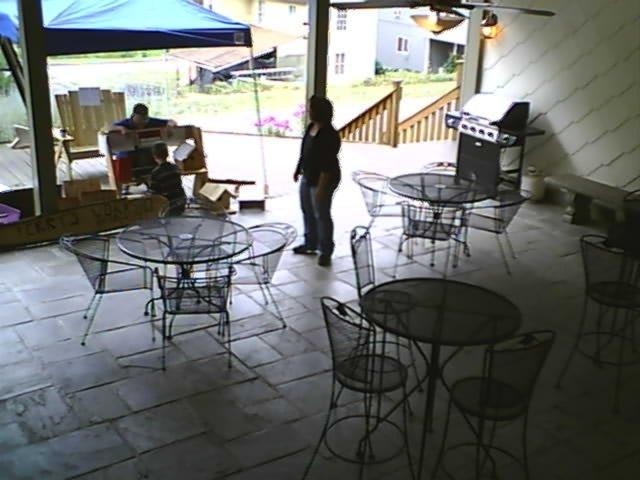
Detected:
[115,214,255,342]
[357,275,526,477]
[387,170,476,266]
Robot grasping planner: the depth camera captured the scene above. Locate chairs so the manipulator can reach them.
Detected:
[460,186,539,278]
[554,232,638,415]
[159,201,241,307]
[330,225,427,420]
[61,233,160,347]
[421,160,477,246]
[301,295,420,480]
[390,199,473,282]
[152,265,239,374]
[209,220,297,339]
[432,327,560,479]
[351,168,424,255]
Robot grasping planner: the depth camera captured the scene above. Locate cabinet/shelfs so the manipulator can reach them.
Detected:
[98,125,208,199]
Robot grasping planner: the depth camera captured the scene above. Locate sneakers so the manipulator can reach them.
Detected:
[316,246,332,267]
[292,244,319,256]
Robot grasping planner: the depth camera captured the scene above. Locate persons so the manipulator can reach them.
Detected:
[293,95,342,266]
[100,102,177,196]
[145,142,186,211]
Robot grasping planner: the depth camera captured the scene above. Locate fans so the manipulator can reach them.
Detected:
[328,0,557,20]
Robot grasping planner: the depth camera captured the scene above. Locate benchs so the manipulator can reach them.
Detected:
[542,169,640,231]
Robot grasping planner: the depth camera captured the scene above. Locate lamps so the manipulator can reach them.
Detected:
[480,11,498,40]
[410,9,464,35]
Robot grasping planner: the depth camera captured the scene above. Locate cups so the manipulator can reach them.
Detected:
[59,127,67,138]
